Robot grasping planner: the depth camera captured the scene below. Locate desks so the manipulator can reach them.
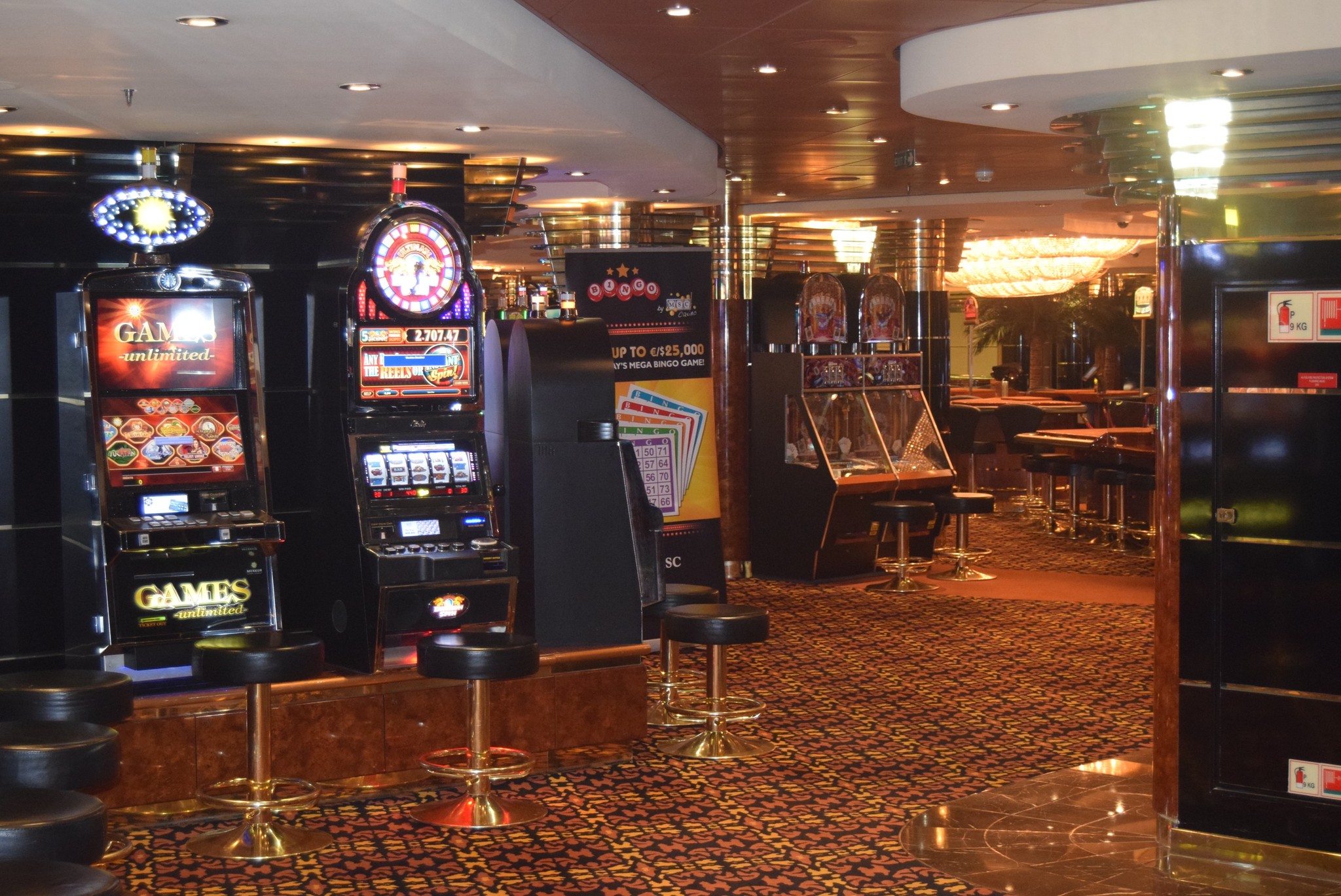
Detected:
[1014,427,1156,520]
[952,395,1088,453]
[1037,388,1140,426]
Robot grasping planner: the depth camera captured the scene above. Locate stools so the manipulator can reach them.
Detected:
[1020,455,1157,552]
[927,492,997,580]
[409,631,549,828]
[866,501,939,592]
[645,582,776,759]
[186,634,333,859]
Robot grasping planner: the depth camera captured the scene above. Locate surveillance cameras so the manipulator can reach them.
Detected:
[1123,214,1132,228]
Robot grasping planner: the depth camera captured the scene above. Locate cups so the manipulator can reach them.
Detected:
[531,294,546,318]
[559,291,575,317]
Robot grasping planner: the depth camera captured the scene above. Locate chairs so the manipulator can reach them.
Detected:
[997,404,1053,502]
[949,405,997,492]
[0,672,135,896]
